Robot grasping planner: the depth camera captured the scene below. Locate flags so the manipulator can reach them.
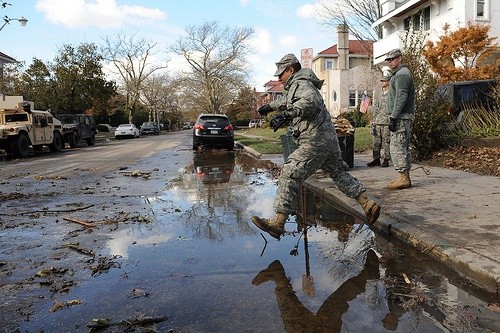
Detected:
[360,94,370,113]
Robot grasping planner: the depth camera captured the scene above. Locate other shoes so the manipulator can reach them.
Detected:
[367,158,381,167]
[381,159,390,167]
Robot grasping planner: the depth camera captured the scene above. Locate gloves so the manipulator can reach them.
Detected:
[388,117,397,132]
[270,112,293,132]
[371,126,376,135]
[258,103,275,116]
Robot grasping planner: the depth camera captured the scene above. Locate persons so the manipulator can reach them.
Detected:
[252,53,381,240]
[252,250,419,333]
[385,49,415,189]
[367,76,391,166]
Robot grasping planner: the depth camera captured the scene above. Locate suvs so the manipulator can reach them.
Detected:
[55,113,97,148]
[0,94,62,158]
[98,123,117,132]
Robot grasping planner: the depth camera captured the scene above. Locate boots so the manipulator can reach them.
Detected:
[355,248,380,283]
[252,260,291,289]
[250,212,287,240]
[384,171,411,191]
[354,192,381,227]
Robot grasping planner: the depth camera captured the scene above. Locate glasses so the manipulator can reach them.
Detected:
[387,56,399,61]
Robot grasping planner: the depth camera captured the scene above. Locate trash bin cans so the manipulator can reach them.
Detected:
[285,134,298,155]
[279,134,291,164]
[337,135,355,168]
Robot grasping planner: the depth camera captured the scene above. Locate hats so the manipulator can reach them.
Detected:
[385,49,402,61]
[273,53,299,77]
[380,76,391,81]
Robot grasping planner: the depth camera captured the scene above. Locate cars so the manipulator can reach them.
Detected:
[114,124,140,137]
[140,122,159,135]
[193,113,234,150]
